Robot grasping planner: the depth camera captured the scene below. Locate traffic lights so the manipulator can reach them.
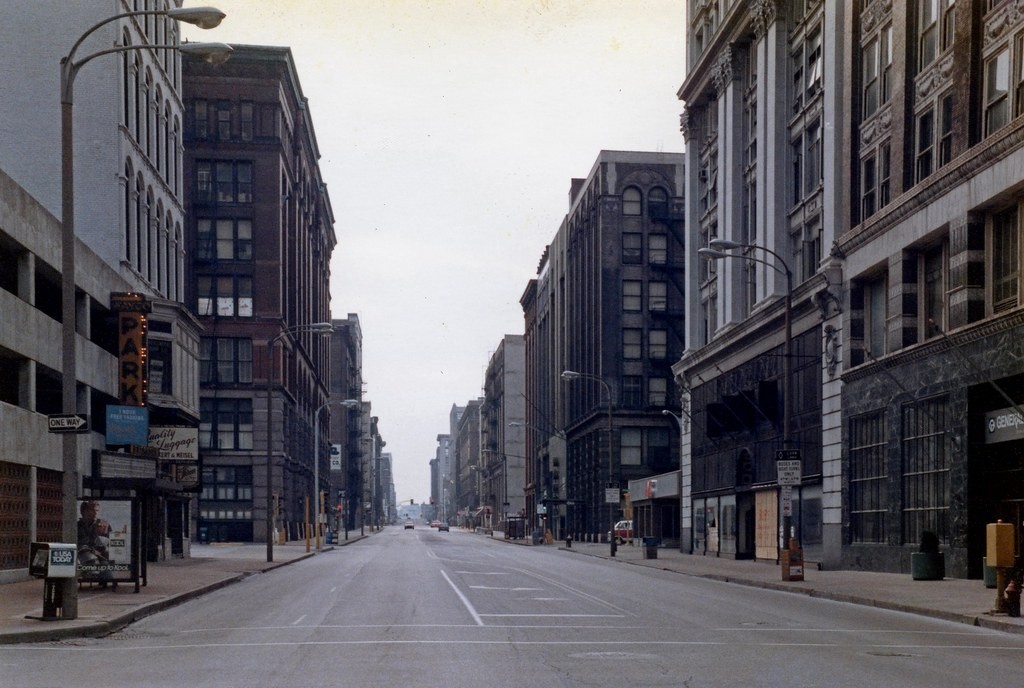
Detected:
[431,500,434,504]
[411,499,413,505]
[542,515,548,519]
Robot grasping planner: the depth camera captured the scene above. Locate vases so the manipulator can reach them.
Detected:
[983,555,1021,588]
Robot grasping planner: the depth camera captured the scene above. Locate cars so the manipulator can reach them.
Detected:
[431,521,440,527]
[438,523,449,532]
[404,522,415,530]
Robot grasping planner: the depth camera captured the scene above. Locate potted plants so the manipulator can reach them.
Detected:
[911,529,946,581]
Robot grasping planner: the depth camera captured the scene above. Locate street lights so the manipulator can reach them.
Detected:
[313,398,359,550]
[508,420,570,500]
[481,449,554,544]
[559,368,619,557]
[265,321,335,562]
[55,5,235,619]
[696,237,795,579]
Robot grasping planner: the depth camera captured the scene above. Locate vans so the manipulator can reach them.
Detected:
[606,520,633,545]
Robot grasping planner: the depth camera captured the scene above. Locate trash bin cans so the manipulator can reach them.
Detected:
[642,536,658,559]
[782,548,804,581]
